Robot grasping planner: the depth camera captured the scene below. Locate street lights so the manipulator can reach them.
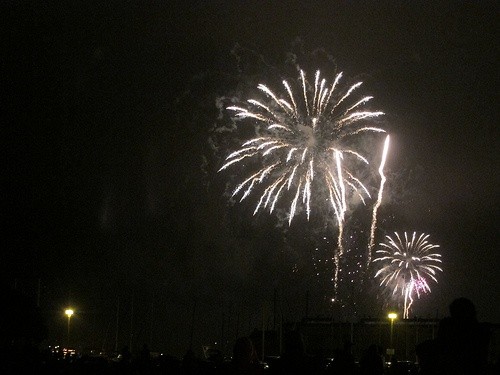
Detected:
[385,308,399,348]
[63,305,76,348]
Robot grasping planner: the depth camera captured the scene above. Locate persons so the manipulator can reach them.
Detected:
[415,297,491,375]
[334,340,355,375]
[418,316,455,375]
[47,341,226,375]
[387,353,402,375]
[358,342,384,375]
[268,330,321,375]
[377,344,388,375]
[221,336,265,375]
